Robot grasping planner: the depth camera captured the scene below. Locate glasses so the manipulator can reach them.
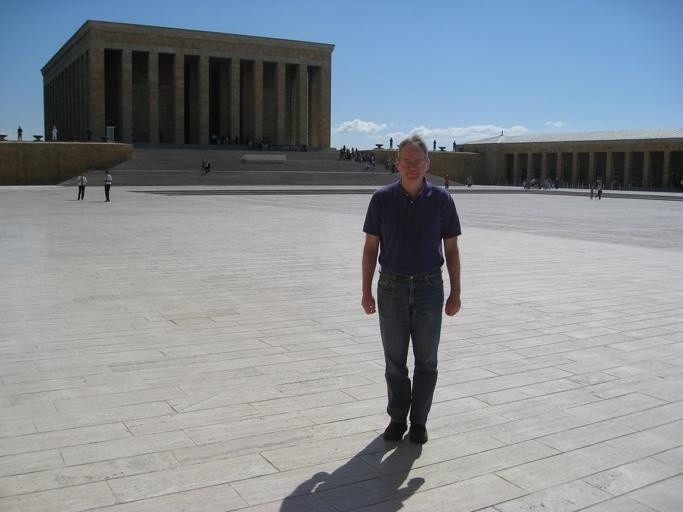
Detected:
[398,158,428,168]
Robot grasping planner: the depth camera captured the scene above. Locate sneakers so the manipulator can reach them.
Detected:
[408,421,429,444]
[383,419,406,441]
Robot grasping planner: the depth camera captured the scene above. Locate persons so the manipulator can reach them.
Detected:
[17,125,22,142]
[76,171,87,200]
[198,158,206,176]
[588,179,595,199]
[452,141,456,152]
[595,180,605,199]
[337,144,398,173]
[204,160,210,172]
[209,130,308,152]
[103,168,112,203]
[51,125,57,142]
[444,172,450,188]
[520,175,562,189]
[360,135,461,446]
[466,174,475,189]
